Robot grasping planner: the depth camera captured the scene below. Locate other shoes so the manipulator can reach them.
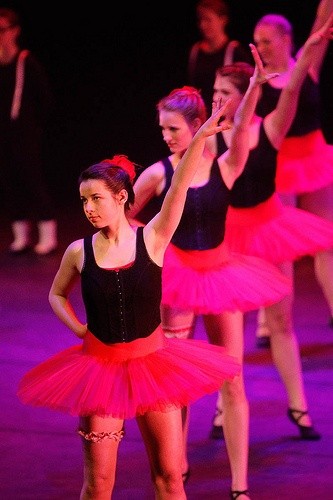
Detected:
[182,466,192,485]
[256,322,270,345]
[230,487,251,500]
[212,406,224,438]
[287,408,321,440]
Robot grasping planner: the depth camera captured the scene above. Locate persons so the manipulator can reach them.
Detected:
[0,9,59,252]
[187,0,333,350]
[124,44,294,500]
[206,14,333,440]
[16,98,242,500]
